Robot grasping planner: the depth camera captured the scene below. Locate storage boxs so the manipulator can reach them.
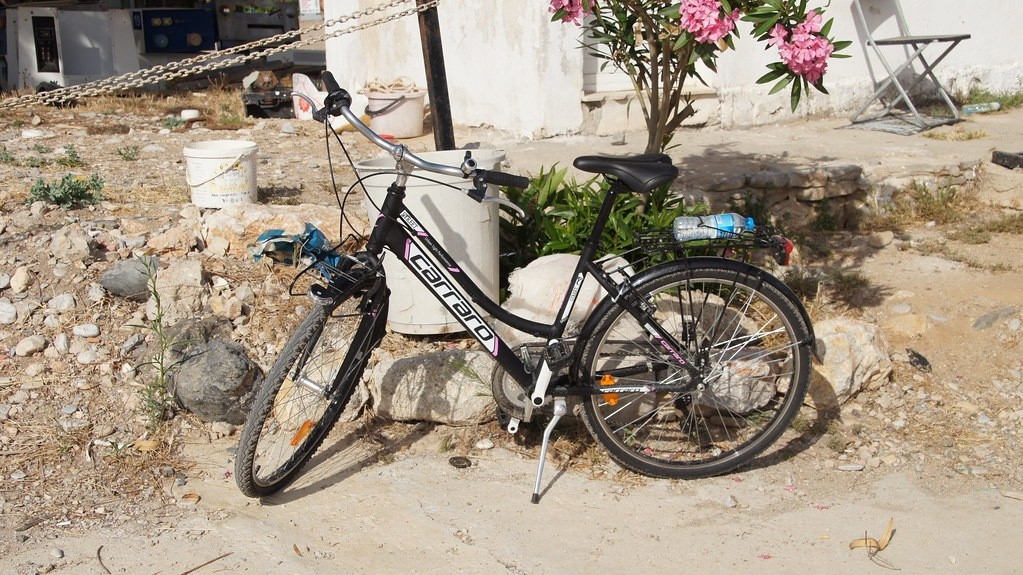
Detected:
[242,86,295,119]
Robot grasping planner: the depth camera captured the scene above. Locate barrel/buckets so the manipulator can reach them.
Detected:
[182,139,260,209]
[364,87,426,139]
[352,146,506,335]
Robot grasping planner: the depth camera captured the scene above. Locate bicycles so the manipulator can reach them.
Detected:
[232,68,825,505]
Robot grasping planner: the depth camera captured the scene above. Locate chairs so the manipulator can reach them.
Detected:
[848,0,972,131]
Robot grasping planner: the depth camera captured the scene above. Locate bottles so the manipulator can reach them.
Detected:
[962,102,1000,113]
[672,212,755,242]
